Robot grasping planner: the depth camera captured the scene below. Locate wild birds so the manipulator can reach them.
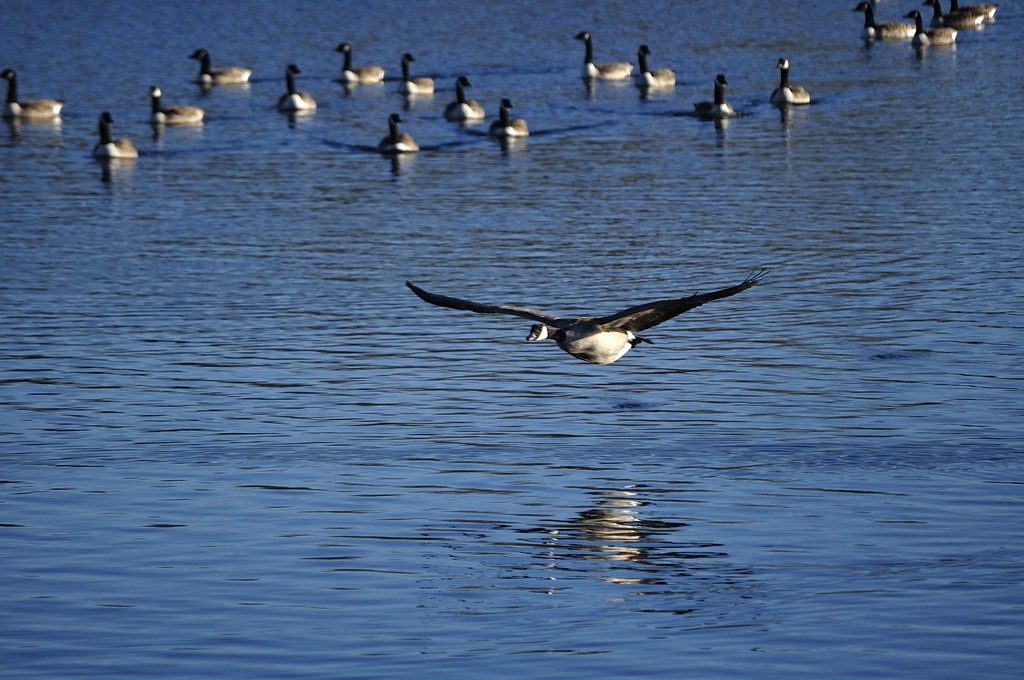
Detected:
[277,63,317,110]
[769,57,811,105]
[633,44,676,86]
[147,85,206,124]
[692,73,735,114]
[921,0,986,28]
[334,42,386,83]
[573,31,634,80]
[397,52,435,94]
[93,112,139,158]
[851,1,917,39]
[378,114,420,153]
[405,268,770,366]
[445,76,486,118]
[0,68,66,119]
[950,0,999,20]
[902,9,958,46]
[489,98,529,136]
[187,48,252,84]
